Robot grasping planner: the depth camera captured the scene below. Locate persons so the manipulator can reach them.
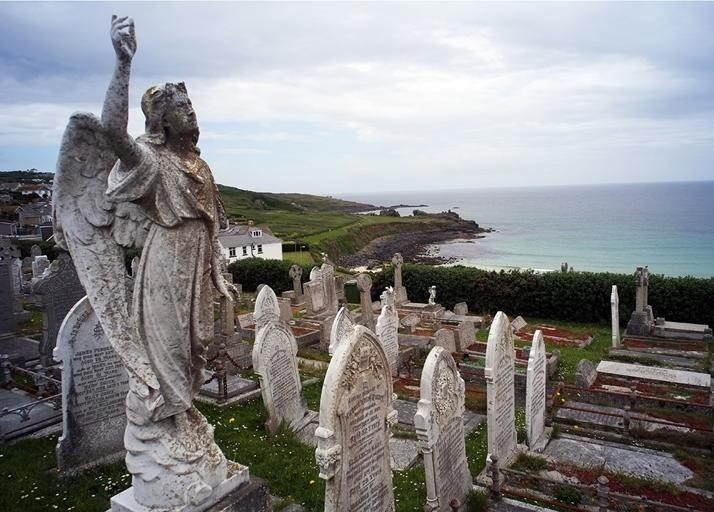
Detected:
[51,16,249,512]
